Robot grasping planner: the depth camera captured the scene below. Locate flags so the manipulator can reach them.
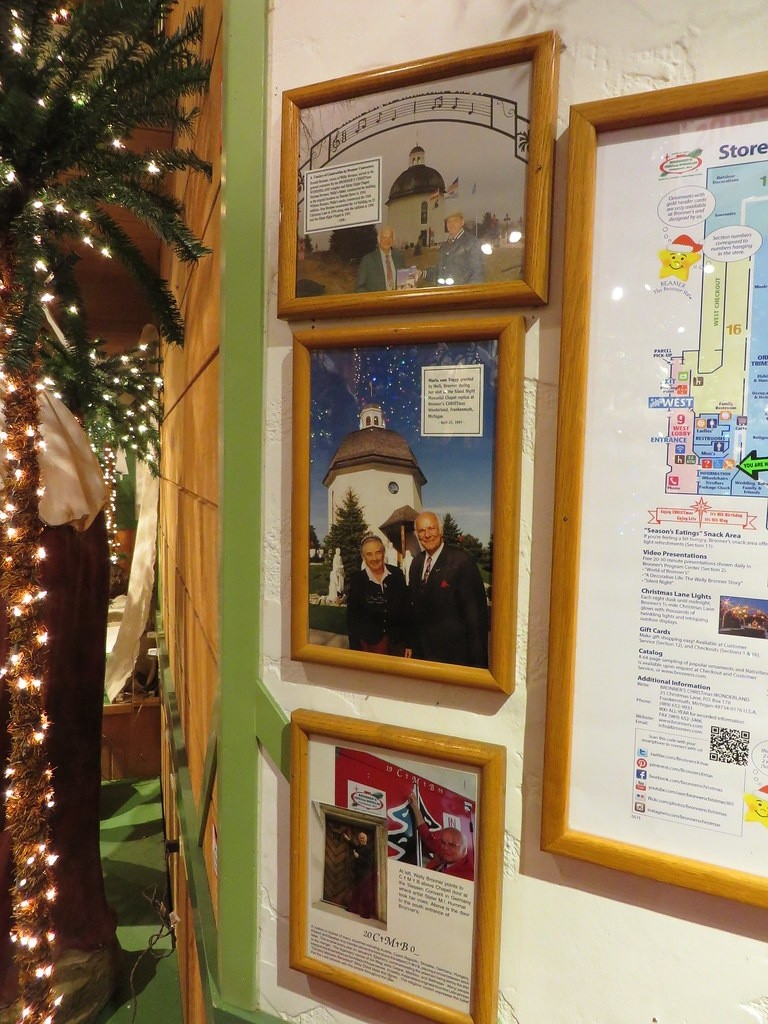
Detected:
[425,185,440,202]
[471,180,478,195]
[444,176,459,198]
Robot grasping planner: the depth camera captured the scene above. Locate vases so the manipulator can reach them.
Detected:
[103,687,161,780]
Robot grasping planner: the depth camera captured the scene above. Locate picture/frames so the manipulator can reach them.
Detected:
[287,705,506,1023]
[289,316,530,698]
[275,26,565,318]
[538,69,768,909]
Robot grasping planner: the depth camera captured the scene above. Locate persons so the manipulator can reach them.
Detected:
[409,787,474,881]
[408,210,484,289]
[345,537,414,660]
[407,511,487,671]
[386,542,413,586]
[355,226,407,294]
[328,547,344,604]
[341,829,374,919]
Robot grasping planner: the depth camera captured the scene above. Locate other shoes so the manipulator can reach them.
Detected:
[345,908,359,914]
[360,914,371,919]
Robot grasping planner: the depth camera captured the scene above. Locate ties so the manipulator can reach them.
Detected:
[385,255,395,290]
[424,557,432,584]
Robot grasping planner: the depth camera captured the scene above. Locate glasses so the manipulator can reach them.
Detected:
[437,839,464,848]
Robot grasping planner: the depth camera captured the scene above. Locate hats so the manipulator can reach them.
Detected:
[444,210,464,222]
